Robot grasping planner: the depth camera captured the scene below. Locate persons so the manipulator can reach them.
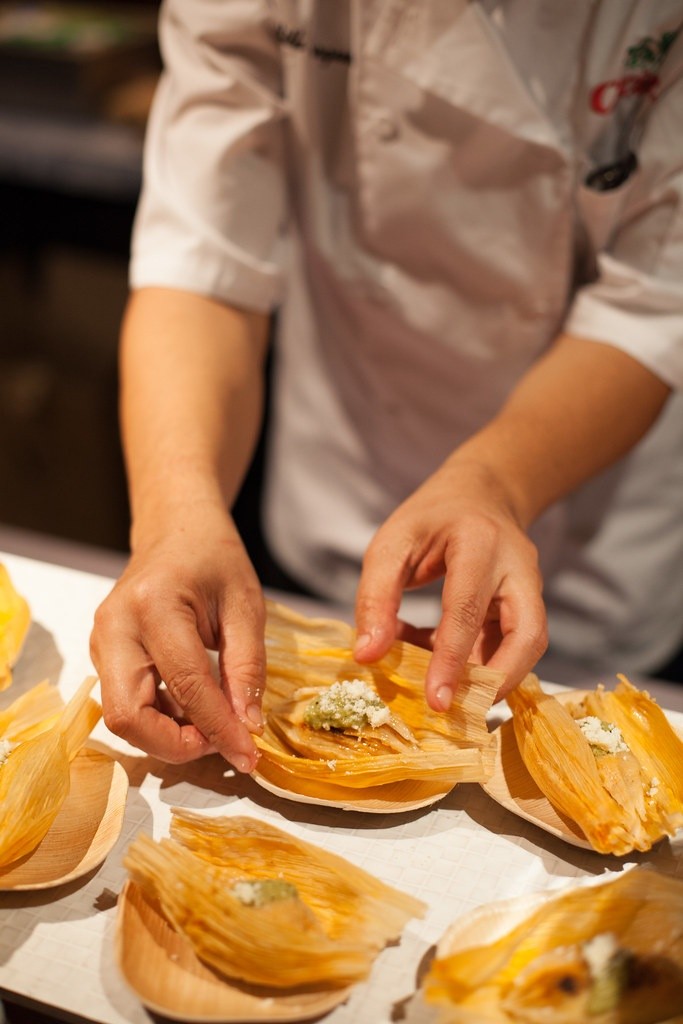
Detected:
[91,0,683,774]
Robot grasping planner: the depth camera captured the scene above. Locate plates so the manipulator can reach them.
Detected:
[0,746,130,891]
[114,874,358,1022]
[478,689,683,850]
[436,888,617,975]
[250,755,457,814]
